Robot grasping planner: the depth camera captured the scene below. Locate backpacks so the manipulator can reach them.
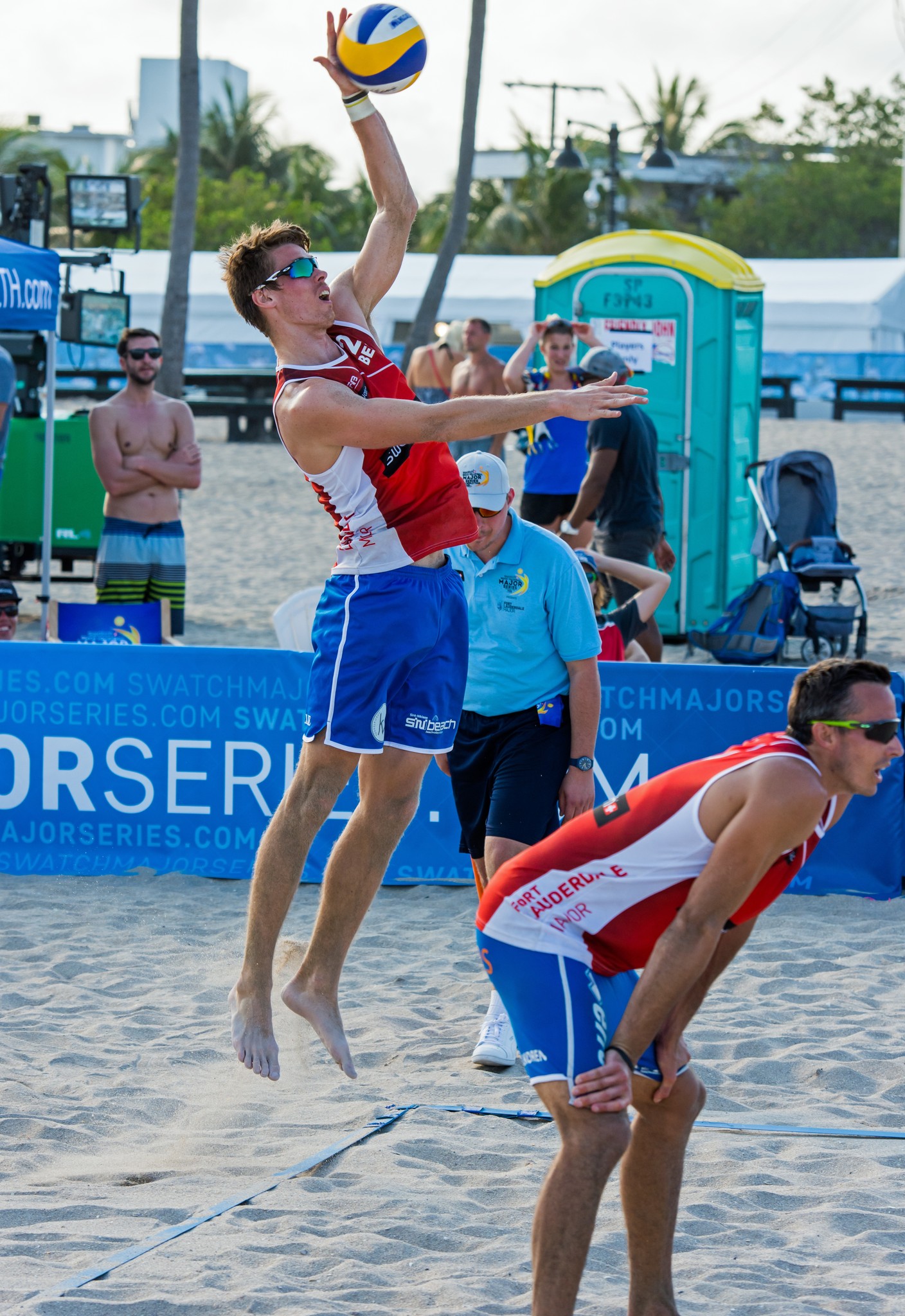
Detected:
[689,570,799,665]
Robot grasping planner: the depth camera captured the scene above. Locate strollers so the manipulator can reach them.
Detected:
[744,448,869,665]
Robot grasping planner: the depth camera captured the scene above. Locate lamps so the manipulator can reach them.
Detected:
[60,262,130,347]
[66,174,147,255]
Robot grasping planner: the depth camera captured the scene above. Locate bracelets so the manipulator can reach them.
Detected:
[604,1045,635,1073]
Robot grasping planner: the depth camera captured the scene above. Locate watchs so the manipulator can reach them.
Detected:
[568,756,594,771]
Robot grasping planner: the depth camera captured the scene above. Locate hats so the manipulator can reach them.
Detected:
[563,346,629,383]
[0,580,22,603]
[574,550,599,576]
[456,450,510,512]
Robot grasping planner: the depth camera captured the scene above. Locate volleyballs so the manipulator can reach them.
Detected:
[336,3,427,95]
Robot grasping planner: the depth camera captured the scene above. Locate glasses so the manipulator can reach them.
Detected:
[544,320,573,331]
[127,348,162,360]
[809,717,901,745]
[248,256,319,296]
[0,605,18,618]
[585,572,598,583]
[473,506,502,518]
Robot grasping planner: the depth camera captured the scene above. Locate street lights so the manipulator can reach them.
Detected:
[543,119,675,236]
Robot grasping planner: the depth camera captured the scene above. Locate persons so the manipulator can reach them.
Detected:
[218,8,647,1081]
[406,315,676,660]
[89,328,202,635]
[0,580,22,640]
[433,450,603,1067]
[475,658,905,1316]
[0,345,16,480]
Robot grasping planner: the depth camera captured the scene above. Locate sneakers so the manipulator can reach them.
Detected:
[471,1012,518,1066]
